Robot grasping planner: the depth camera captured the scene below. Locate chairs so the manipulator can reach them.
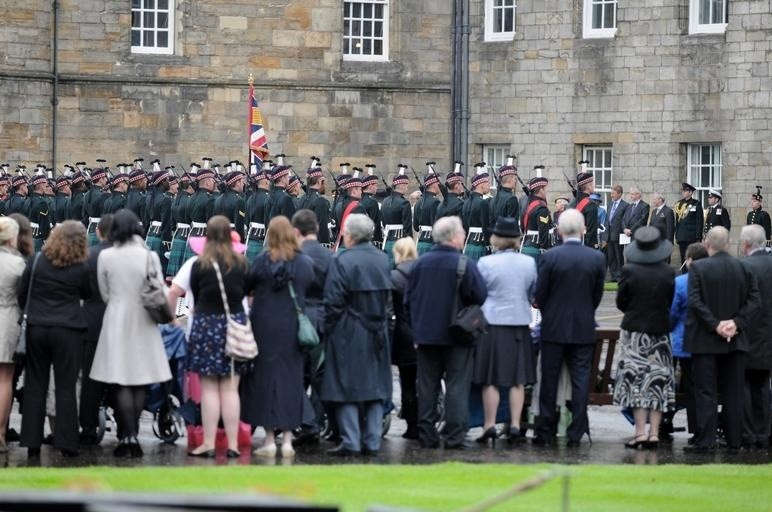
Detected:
[587,329,621,406]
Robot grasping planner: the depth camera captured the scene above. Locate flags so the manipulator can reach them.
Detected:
[250,94,269,178]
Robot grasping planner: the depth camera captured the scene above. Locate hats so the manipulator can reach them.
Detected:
[422,165,517,188]
[751,194,762,201]
[489,217,521,237]
[681,184,722,196]
[188,231,247,255]
[528,178,548,190]
[391,175,409,185]
[625,224,676,266]
[555,195,572,203]
[336,176,377,188]
[588,194,602,202]
[576,172,593,185]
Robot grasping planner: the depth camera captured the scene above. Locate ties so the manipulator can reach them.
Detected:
[630,203,635,217]
[651,209,656,222]
[610,203,616,220]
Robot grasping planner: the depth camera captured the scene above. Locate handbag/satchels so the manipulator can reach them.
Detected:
[143,275,173,325]
[16,319,27,355]
[223,318,260,361]
[453,303,489,351]
[296,312,323,348]
[390,314,412,365]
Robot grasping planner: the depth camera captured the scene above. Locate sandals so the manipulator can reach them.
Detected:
[626,434,659,450]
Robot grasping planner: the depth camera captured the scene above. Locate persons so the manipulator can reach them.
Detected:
[612,225,772,459]
[1,207,607,460]
[552,183,771,283]
[1,169,598,313]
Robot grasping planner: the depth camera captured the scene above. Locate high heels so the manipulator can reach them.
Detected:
[254,444,277,458]
[476,426,496,445]
[282,445,297,458]
[509,428,520,448]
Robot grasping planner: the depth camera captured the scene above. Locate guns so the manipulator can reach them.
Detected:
[139,165,151,184]
[41,168,55,188]
[243,165,258,193]
[516,174,531,197]
[180,165,197,192]
[491,166,501,184]
[213,167,230,194]
[410,167,426,194]
[76,164,115,193]
[291,168,306,192]
[2,166,15,196]
[173,168,189,190]
[56,167,72,187]
[379,172,393,195]
[430,164,449,200]
[462,183,470,198]
[327,167,339,186]
[18,167,35,194]
[563,172,604,230]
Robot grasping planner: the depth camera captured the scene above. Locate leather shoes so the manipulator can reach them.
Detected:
[682,443,715,455]
[187,449,216,459]
[533,435,551,447]
[325,443,379,459]
[27,448,41,460]
[114,435,144,459]
[567,440,580,447]
[226,450,242,458]
